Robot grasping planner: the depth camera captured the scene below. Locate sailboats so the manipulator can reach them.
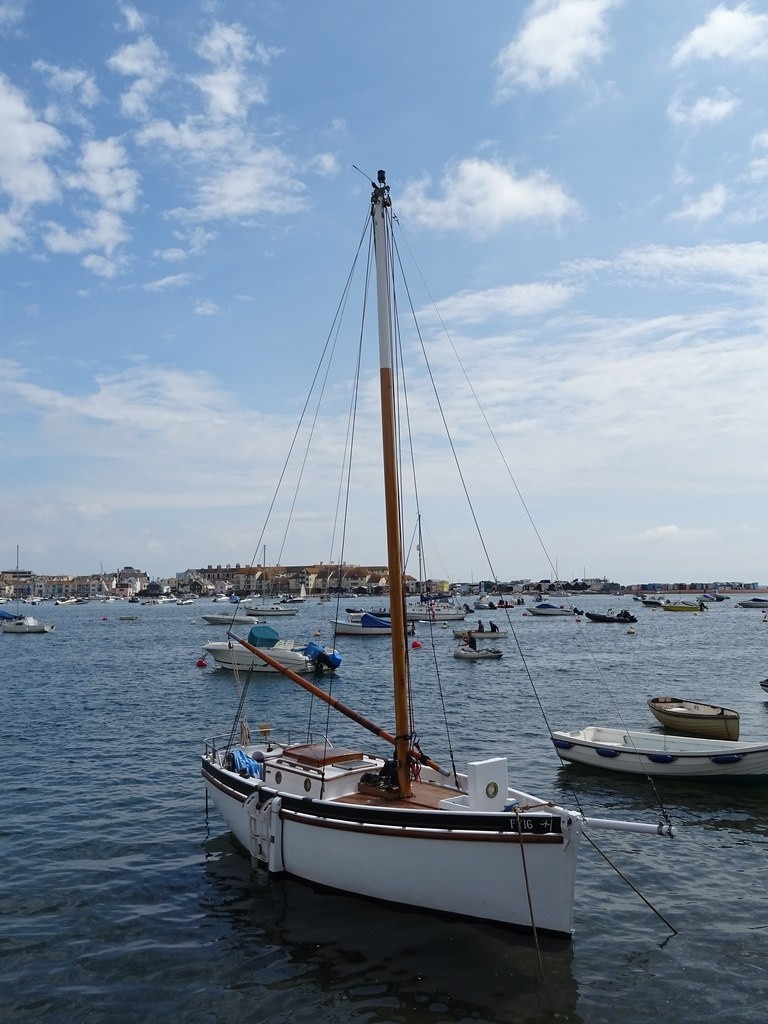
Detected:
[199,162,679,940]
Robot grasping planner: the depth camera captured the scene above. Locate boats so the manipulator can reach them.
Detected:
[552,726,768,782]
[471,588,573,610]
[452,629,508,639]
[390,514,466,620]
[202,626,342,673]
[611,588,768,611]
[344,607,391,617]
[454,649,503,660]
[525,603,585,617]
[330,612,416,635]
[585,608,639,623]
[647,696,740,740]
[0,580,335,633]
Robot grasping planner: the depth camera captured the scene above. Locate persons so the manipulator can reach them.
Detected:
[475,620,485,632]
[458,631,476,652]
[489,621,499,632]
[428,603,435,622]
